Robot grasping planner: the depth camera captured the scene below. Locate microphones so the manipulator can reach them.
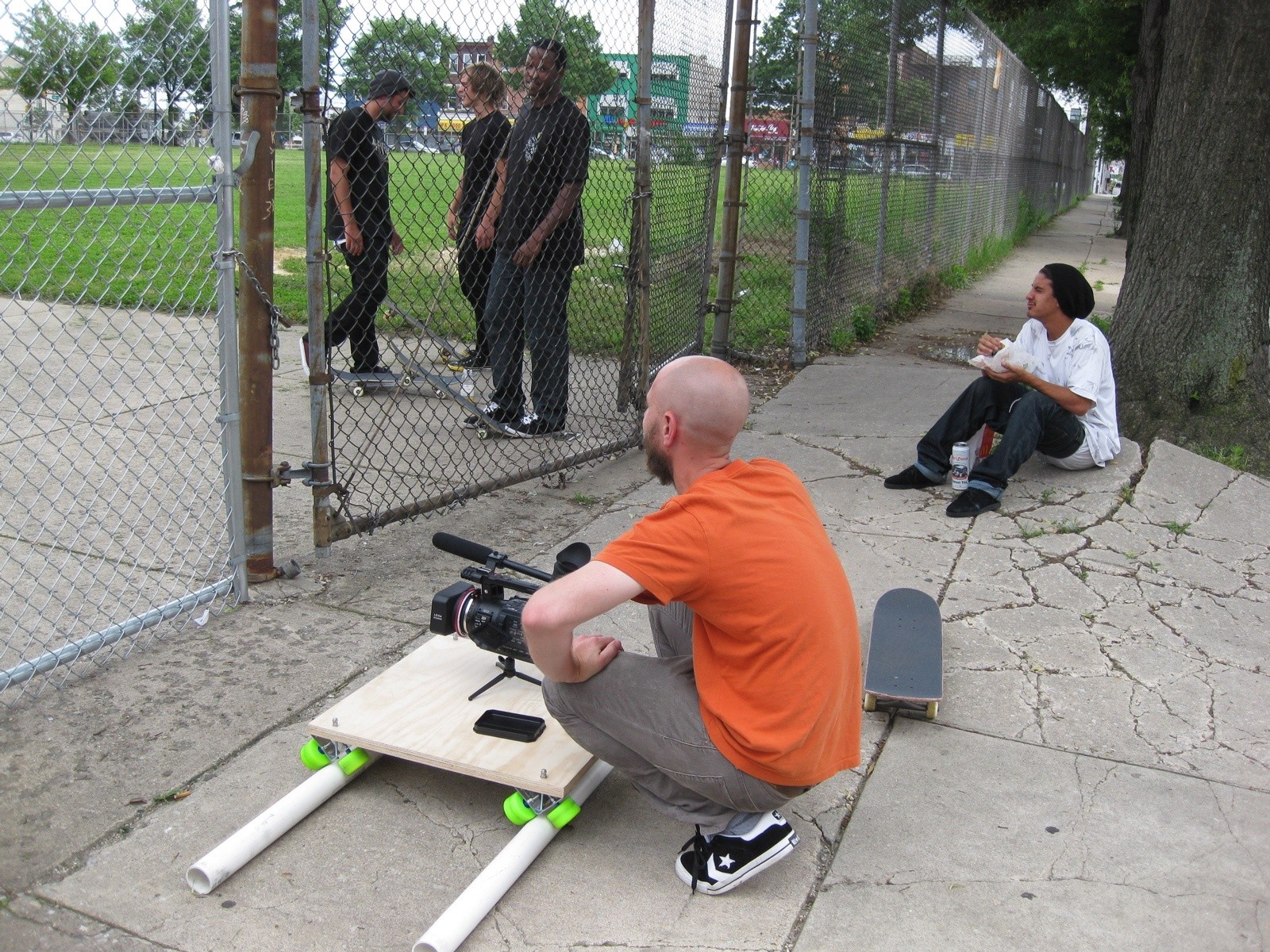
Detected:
[431,533,551,581]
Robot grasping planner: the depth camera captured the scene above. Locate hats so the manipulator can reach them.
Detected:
[1044,263,1095,319]
[366,68,412,100]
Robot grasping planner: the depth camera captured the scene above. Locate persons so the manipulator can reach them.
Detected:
[519,354,863,896]
[447,61,514,371]
[883,264,1121,516]
[461,37,590,440]
[298,69,416,383]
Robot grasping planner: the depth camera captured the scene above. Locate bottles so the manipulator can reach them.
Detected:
[460,369,474,404]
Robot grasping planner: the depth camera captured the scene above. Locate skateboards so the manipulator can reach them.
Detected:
[387,334,519,439]
[326,368,478,398]
[382,298,466,364]
[864,588,944,720]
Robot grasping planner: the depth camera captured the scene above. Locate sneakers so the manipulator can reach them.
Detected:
[505,412,566,438]
[463,401,525,429]
[447,350,492,370]
[675,808,799,895]
[461,349,477,357]
[884,465,942,489]
[367,367,390,374]
[300,337,310,375]
[946,487,1001,517]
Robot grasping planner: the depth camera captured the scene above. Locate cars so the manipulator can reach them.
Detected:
[211,132,241,147]
[284,132,327,150]
[647,145,967,182]
[386,141,440,154]
[588,147,620,161]
[0,131,26,144]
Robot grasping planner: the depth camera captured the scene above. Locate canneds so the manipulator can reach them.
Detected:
[952,442,970,490]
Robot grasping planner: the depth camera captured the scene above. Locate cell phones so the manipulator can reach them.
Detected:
[475,710,546,742]
